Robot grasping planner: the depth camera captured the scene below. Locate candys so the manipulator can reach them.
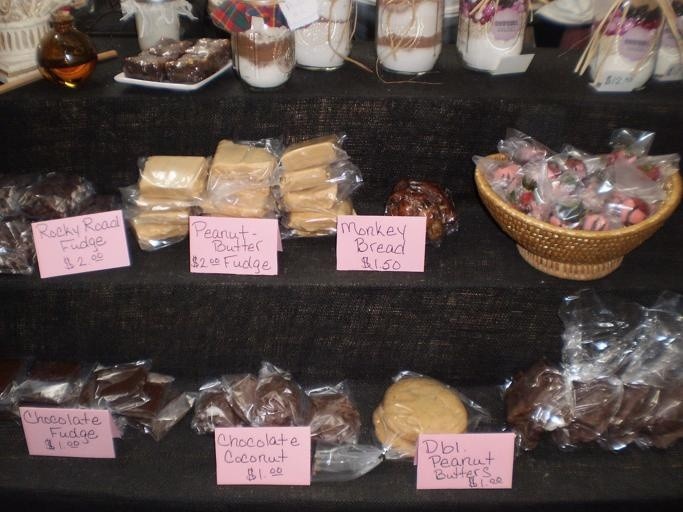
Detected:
[493,148,660,229]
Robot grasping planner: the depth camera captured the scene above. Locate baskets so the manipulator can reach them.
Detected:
[472,153,683,280]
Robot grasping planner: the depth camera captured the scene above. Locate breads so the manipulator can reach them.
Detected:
[506,358,683,448]
[132,135,353,242]
[386,179,456,240]
[0,170,117,275]
[125,36,229,83]
[18,364,164,420]
[196,377,359,444]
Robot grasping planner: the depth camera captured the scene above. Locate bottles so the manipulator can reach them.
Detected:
[33,12,98,90]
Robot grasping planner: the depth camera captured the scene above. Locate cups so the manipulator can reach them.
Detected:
[134,6,182,52]
[287,2,352,73]
[374,0,445,76]
[229,26,294,91]
[457,0,527,72]
[587,1,683,92]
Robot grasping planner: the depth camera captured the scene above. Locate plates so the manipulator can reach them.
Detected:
[110,59,234,95]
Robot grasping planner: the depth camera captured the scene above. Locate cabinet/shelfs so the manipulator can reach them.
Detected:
[0,0,683,512]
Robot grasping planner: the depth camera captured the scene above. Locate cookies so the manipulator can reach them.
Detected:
[373,379,469,448]
